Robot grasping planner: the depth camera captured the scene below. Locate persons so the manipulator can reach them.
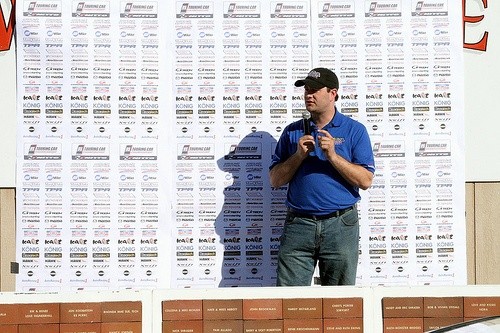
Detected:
[270,69,376,287]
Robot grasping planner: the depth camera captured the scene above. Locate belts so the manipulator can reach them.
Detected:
[287,207,354,218]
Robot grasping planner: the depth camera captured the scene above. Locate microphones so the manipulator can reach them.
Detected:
[302,110,312,148]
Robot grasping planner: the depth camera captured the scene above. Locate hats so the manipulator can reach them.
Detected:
[295,67,339,88]
